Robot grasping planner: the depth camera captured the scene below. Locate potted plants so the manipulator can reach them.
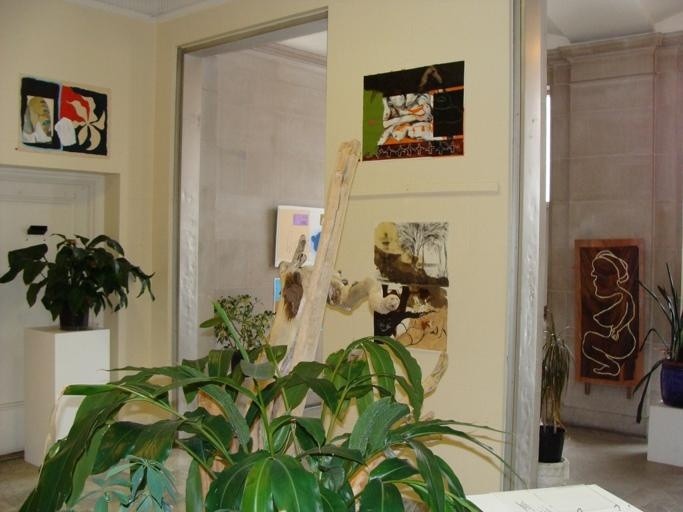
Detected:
[12,344,531,510]
[539,309,572,463]
[209,293,278,375]
[0,223,157,329]
[629,261,683,423]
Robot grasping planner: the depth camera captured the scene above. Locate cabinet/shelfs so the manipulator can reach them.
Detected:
[21,322,111,467]
[646,402,683,468]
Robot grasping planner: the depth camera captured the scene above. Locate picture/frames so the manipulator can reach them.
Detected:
[573,238,646,399]
[14,68,115,161]
